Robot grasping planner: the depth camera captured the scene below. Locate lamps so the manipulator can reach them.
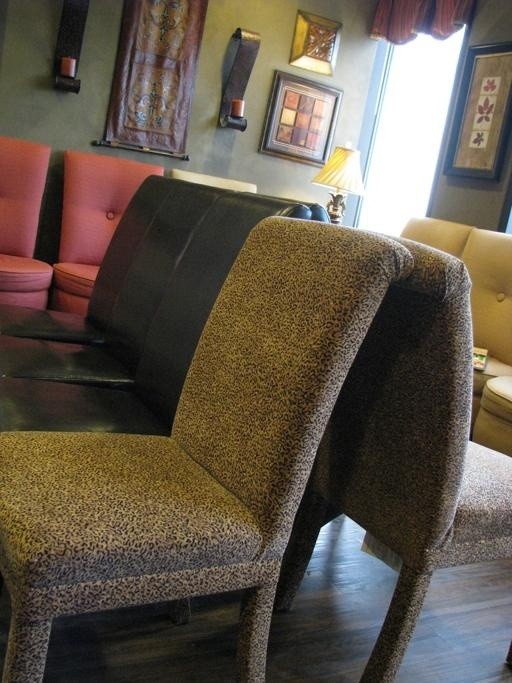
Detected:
[309,143,369,228]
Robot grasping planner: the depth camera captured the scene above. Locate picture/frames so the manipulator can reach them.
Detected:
[259,69,345,168]
[440,43,512,181]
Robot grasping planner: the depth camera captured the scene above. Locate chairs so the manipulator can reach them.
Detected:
[286,218,510,679]
[1,138,56,311]
[3,212,418,683]
[0,190,317,436]
[396,205,512,459]
[51,146,167,312]
[0,171,229,383]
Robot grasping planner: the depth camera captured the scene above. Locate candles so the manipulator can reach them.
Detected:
[231,98,244,118]
[58,55,76,77]
